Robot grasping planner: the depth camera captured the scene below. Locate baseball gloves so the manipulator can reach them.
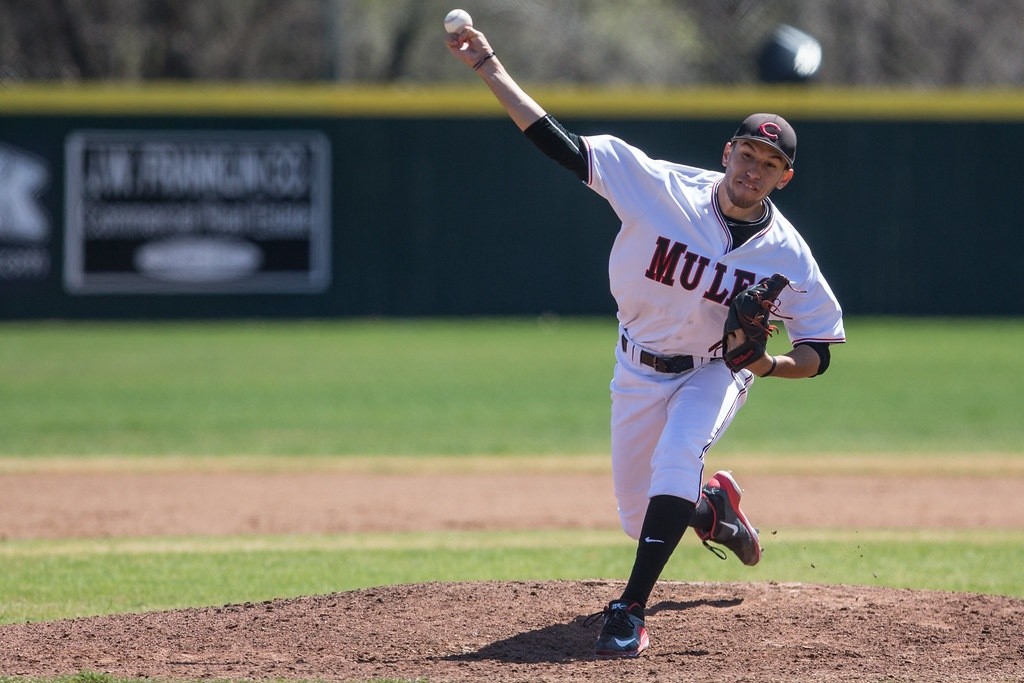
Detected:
[722,273,790,374]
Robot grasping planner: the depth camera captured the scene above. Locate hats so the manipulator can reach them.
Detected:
[732,113,796,168]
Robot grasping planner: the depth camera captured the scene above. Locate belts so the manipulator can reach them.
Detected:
[622,334,715,374]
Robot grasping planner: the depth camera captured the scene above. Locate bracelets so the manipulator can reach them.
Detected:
[760,356,777,377]
[473,51,496,72]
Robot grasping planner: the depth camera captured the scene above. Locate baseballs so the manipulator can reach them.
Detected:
[444,9,473,34]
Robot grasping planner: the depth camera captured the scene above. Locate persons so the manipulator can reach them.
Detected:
[445,25,845,656]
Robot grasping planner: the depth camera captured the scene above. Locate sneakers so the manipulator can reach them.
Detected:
[595,600,649,657]
[694,470,760,566]
